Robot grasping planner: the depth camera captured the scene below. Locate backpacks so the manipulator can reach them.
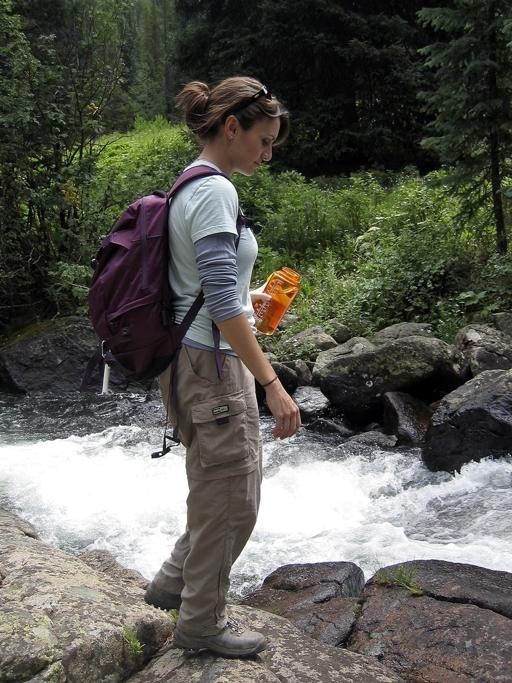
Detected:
[88,165,254,383]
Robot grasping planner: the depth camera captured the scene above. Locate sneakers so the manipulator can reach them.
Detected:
[144,581,267,657]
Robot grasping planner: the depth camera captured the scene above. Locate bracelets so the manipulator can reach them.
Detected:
[261,373,279,389]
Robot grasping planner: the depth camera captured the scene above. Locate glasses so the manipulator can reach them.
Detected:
[240,84,271,114]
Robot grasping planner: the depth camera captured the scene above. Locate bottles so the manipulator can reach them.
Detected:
[252,267,303,335]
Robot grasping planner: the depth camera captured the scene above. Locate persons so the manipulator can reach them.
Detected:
[144,73,303,656]
